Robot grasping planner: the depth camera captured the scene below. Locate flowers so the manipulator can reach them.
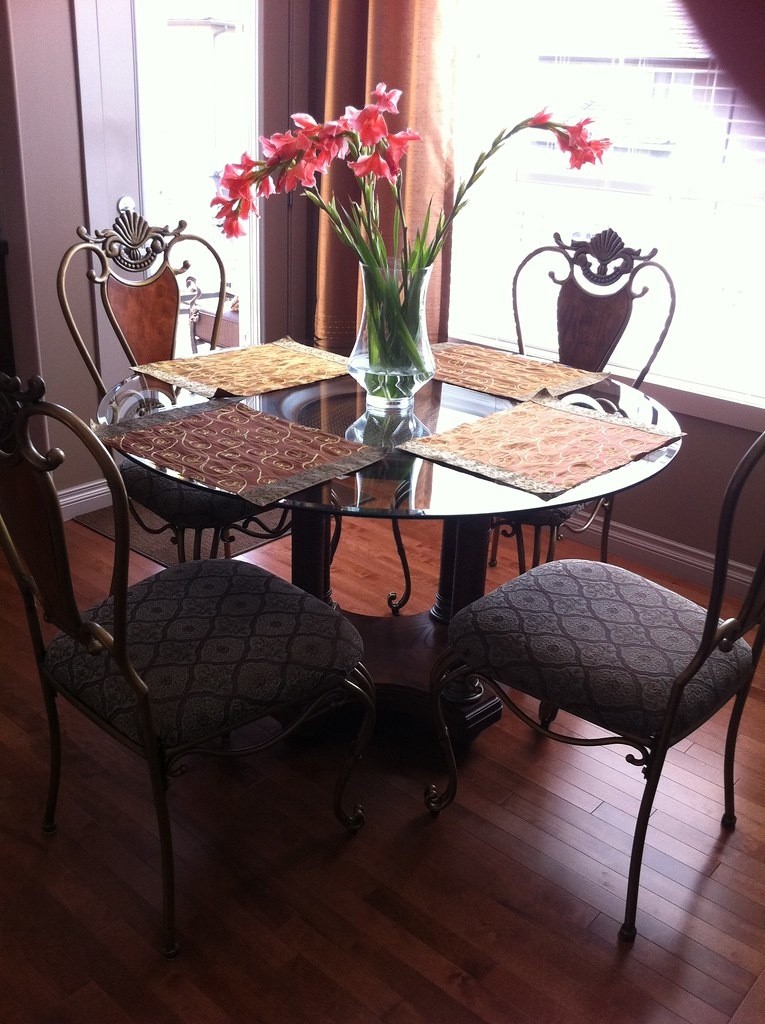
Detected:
[210,82,613,396]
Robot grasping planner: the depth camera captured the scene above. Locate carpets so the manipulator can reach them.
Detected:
[73,481,374,569]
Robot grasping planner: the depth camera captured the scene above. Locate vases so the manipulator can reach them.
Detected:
[345,257,437,449]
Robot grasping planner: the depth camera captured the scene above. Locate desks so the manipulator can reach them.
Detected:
[97,345,686,748]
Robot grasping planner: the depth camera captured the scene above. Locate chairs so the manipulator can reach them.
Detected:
[489,228,677,574]
[58,208,342,564]
[187,276,240,355]
[0,372,373,958]
[427,430,765,946]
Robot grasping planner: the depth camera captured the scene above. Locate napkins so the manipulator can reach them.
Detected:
[129,334,351,402]
[429,338,610,401]
[397,398,688,494]
[90,397,390,506]
